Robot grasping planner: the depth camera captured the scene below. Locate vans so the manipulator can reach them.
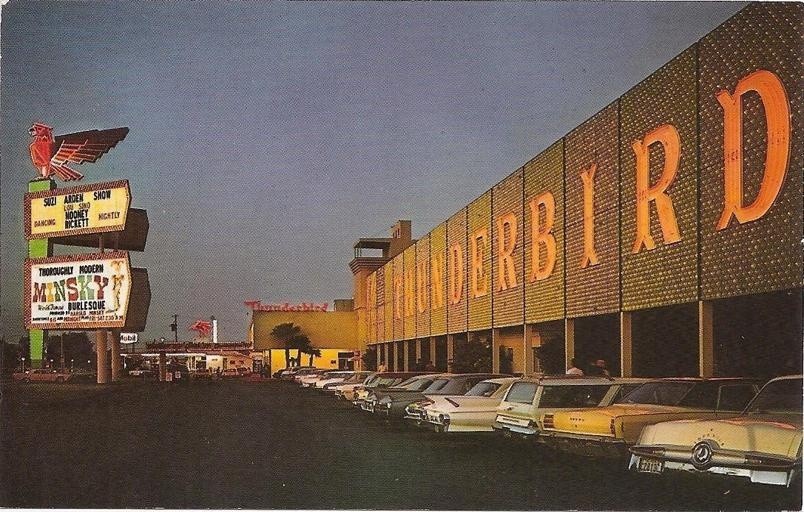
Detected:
[496,374,648,447]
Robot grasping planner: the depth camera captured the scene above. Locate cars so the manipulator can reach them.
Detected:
[619,371,802,497]
[535,371,761,464]
[10,356,187,384]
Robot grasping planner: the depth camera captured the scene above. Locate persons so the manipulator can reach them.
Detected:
[566,357,584,375]
[378,361,388,372]
[595,359,610,376]
[416,358,425,371]
[425,360,437,371]
